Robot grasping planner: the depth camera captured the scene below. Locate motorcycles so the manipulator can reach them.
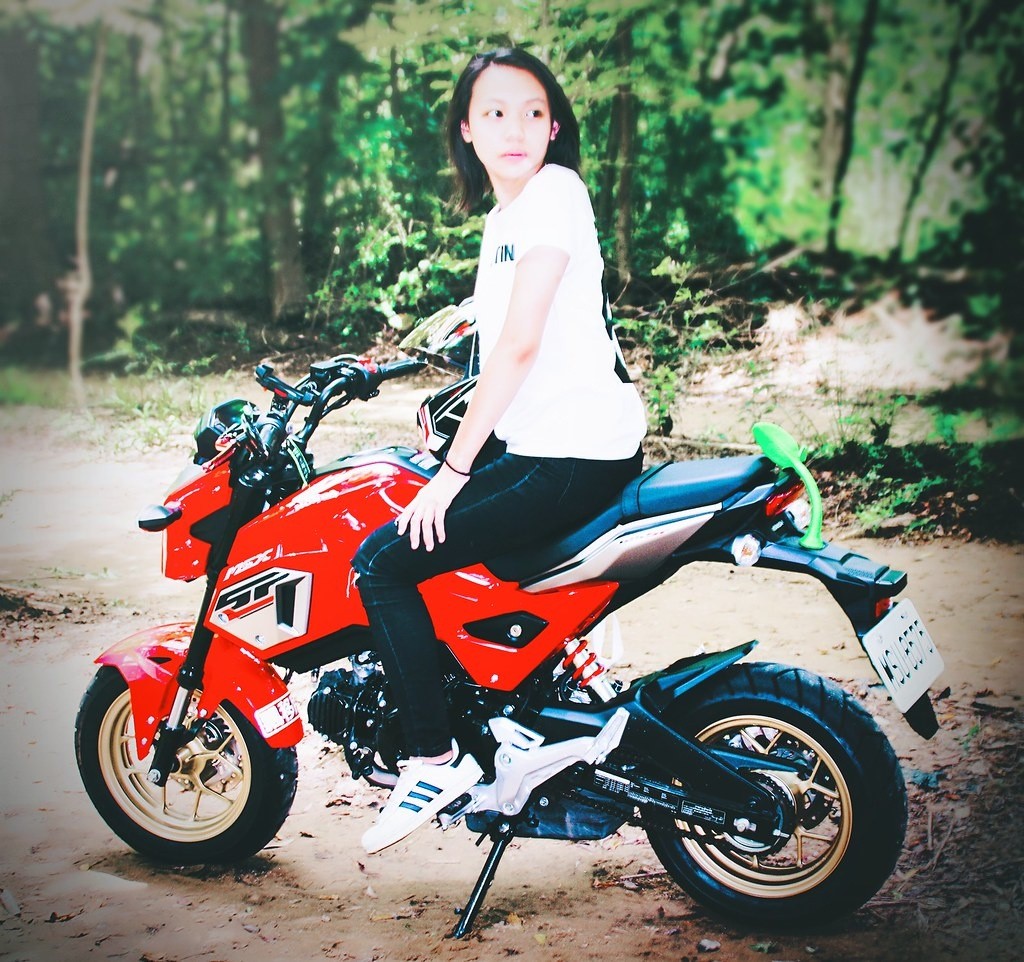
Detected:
[73,296,947,941]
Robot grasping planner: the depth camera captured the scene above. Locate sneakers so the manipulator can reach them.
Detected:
[362,736,483,854]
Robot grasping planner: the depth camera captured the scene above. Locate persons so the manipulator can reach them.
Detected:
[350,45,647,855]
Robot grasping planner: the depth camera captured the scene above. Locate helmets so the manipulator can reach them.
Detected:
[399,294,507,470]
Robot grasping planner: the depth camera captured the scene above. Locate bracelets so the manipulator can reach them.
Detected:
[444,457,470,477]
